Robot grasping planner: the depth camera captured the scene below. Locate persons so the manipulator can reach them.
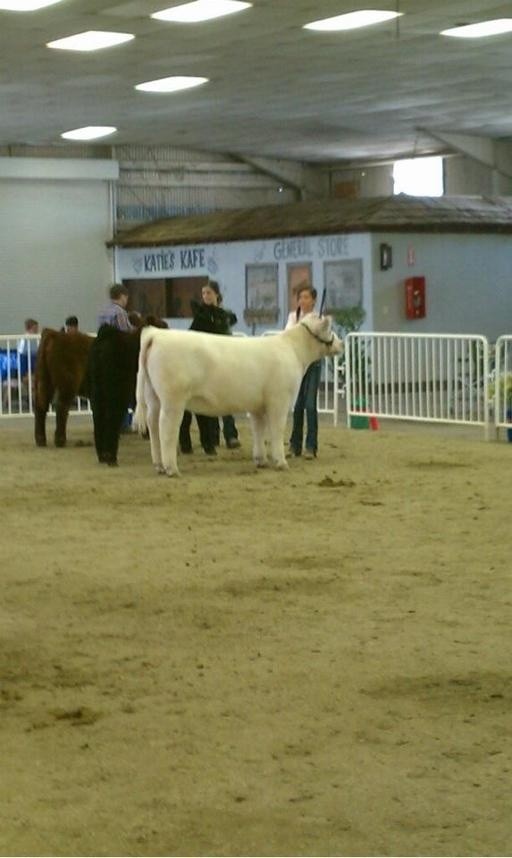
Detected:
[126,306,145,332]
[95,282,133,432]
[283,281,326,460]
[196,278,242,450]
[57,315,78,334]
[17,316,42,352]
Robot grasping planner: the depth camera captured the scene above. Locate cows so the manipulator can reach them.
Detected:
[131,310,345,478]
[34,309,170,447]
[85,298,233,468]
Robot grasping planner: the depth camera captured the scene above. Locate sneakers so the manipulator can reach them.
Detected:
[284,444,317,461]
[225,436,242,449]
